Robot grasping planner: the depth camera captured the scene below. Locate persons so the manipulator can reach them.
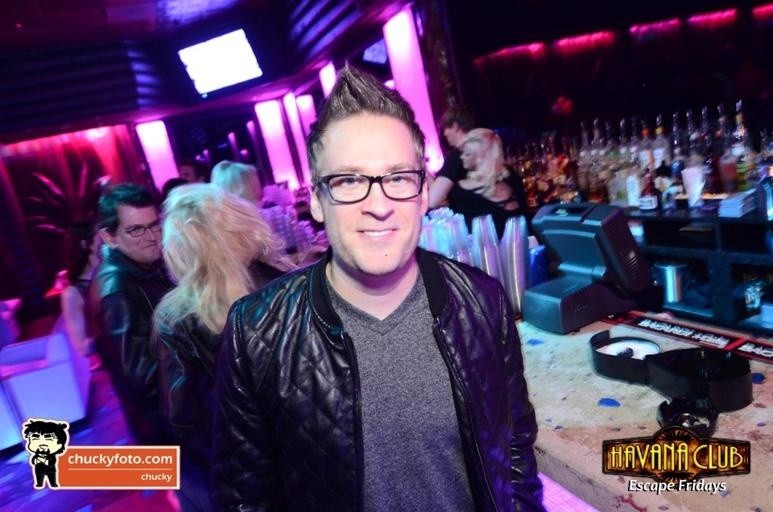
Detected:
[448,127,534,244]
[85,180,176,444]
[212,57,546,511]
[60,219,104,373]
[428,103,476,211]
[213,158,261,204]
[178,159,210,182]
[151,181,303,491]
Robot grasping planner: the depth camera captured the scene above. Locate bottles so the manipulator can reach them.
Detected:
[497,99,773,207]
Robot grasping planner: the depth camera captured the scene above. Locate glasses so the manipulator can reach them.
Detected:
[310,163,431,206]
[113,218,164,239]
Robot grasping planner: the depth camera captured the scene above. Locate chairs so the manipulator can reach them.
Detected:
[0,290,92,450]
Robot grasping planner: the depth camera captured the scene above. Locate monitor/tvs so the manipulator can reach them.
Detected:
[177,28,264,95]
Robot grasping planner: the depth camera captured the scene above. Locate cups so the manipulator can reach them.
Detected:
[258,202,329,263]
[420,205,530,315]
[653,261,688,305]
